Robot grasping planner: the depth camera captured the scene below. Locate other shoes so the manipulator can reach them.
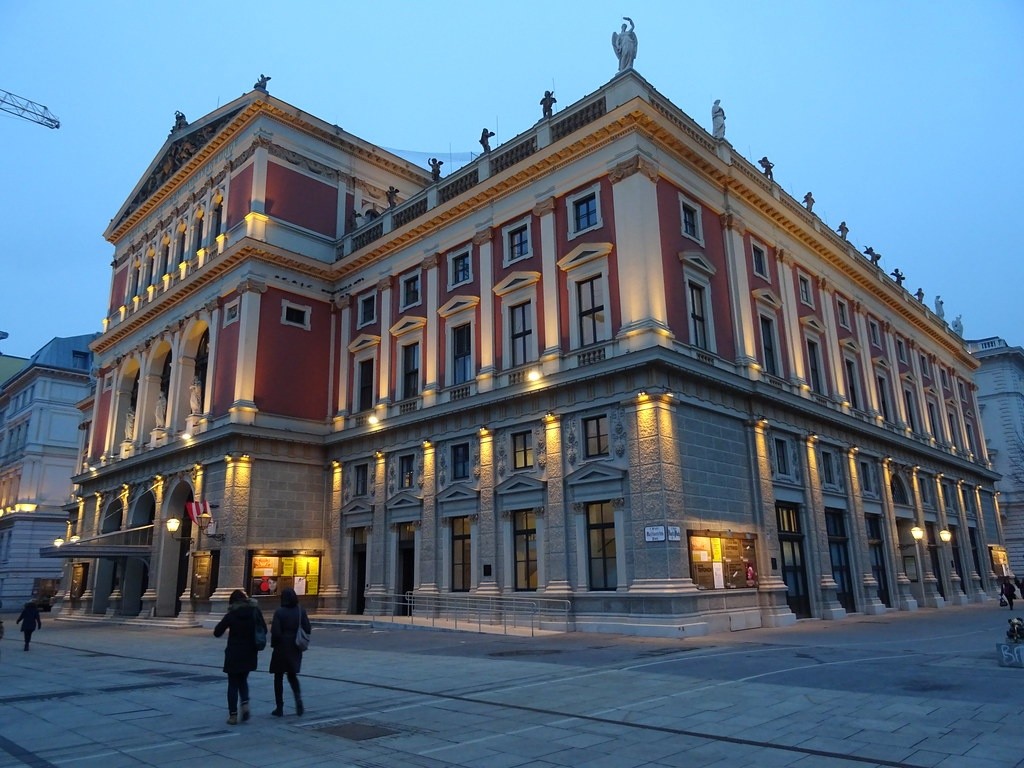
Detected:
[242,704,249,721]
[24,643,29,651]
[297,698,304,716]
[1010,605,1013,610]
[227,715,237,724]
[272,703,284,716]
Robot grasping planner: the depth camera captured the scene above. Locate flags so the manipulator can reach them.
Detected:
[184,498,213,527]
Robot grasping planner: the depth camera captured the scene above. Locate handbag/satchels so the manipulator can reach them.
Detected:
[1000,597,1008,606]
[253,607,266,650]
[296,606,310,651]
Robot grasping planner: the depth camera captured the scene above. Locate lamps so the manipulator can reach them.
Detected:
[926,527,952,550]
[166,515,194,544]
[899,522,923,550]
[197,511,227,542]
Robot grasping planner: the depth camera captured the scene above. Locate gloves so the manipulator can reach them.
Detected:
[17,619,20,624]
[38,622,41,629]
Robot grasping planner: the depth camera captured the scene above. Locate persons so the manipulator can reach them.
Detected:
[803,192,814,212]
[935,296,944,318]
[155,392,166,428]
[386,186,399,207]
[712,100,726,138]
[350,210,362,227]
[258,74,266,89]
[428,158,443,180]
[891,269,905,282]
[952,315,963,337]
[540,91,557,117]
[612,17,637,70]
[999,574,1024,610]
[16,598,42,651]
[269,587,311,717]
[914,288,924,302]
[213,589,268,725]
[189,376,201,413]
[267,576,277,595]
[838,222,848,240]
[124,408,135,439]
[480,128,495,147]
